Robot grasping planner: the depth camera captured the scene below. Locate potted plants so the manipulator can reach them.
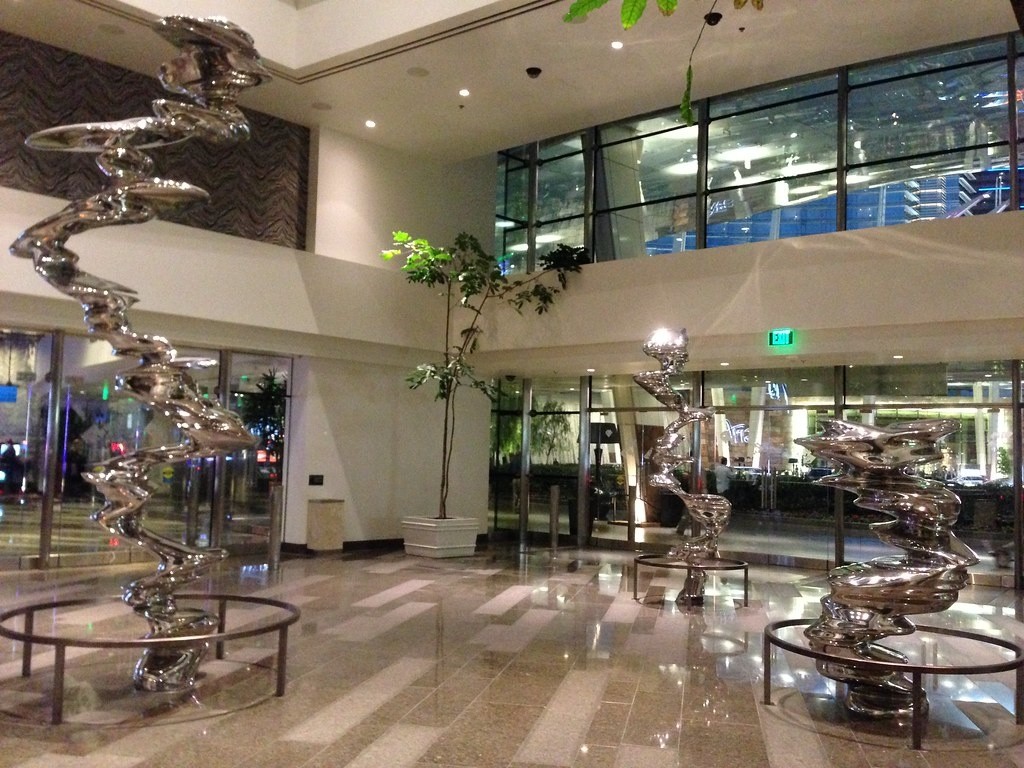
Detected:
[379,230,589,560]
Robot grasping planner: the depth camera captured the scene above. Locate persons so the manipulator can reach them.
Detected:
[1,438,19,495]
[736,457,747,477]
[715,457,736,511]
[60,438,93,503]
